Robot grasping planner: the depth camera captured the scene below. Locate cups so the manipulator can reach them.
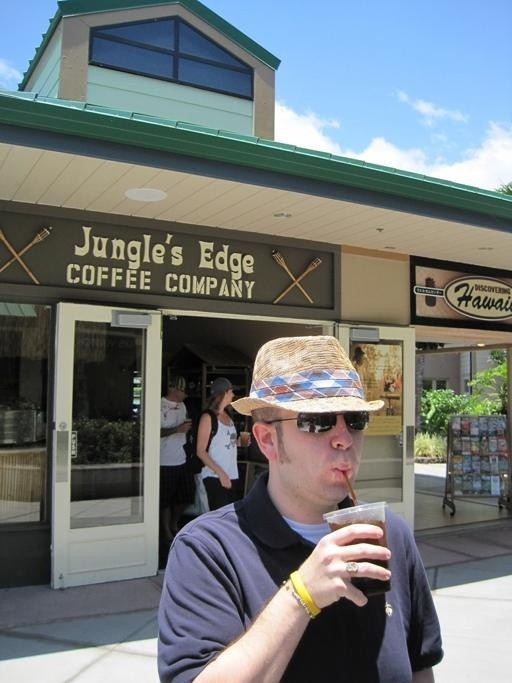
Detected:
[321,501,392,599]
[240,431,250,447]
[184,419,193,425]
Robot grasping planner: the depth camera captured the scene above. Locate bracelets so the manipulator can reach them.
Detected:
[282,581,317,622]
[289,570,321,617]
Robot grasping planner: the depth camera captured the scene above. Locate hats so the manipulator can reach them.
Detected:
[231,336,385,417]
[209,377,231,392]
[168,372,199,393]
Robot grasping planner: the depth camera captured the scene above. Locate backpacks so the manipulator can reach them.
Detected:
[183,409,217,474]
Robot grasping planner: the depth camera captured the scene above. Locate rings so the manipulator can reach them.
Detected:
[346,561,359,576]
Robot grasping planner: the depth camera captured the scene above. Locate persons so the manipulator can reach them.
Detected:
[160,376,193,547]
[196,377,240,511]
[353,346,366,373]
[158,336,443,683]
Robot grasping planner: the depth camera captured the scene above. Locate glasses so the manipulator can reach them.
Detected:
[267,412,369,433]
